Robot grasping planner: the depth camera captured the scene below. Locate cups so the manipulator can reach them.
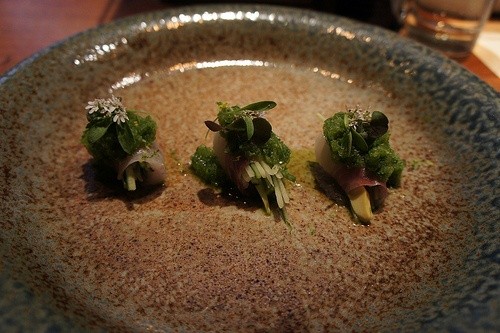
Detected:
[400,1,495,61]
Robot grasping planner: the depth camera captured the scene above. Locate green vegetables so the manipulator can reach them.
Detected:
[82,90,404,222]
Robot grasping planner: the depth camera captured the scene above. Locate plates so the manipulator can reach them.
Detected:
[0,6,500,332]
